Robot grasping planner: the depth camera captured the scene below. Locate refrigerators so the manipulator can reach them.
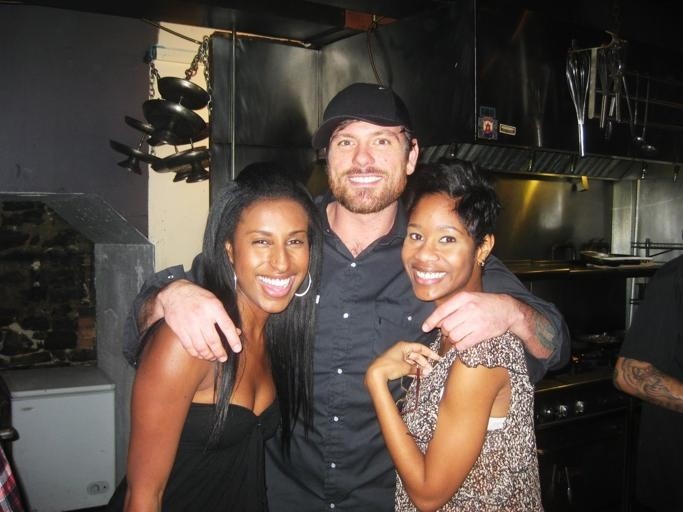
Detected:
[3,364,117,511]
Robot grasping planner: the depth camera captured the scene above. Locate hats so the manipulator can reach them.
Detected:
[311,82,410,151]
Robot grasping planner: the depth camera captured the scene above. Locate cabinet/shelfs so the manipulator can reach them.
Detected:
[500,256,662,511]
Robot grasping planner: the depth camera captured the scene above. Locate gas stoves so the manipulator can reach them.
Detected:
[534,326,640,428]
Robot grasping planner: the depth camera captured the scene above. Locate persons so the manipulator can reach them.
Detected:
[360,155,546,511]
[102,162,326,512]
[120,82,574,512]
[612,254,683,511]
